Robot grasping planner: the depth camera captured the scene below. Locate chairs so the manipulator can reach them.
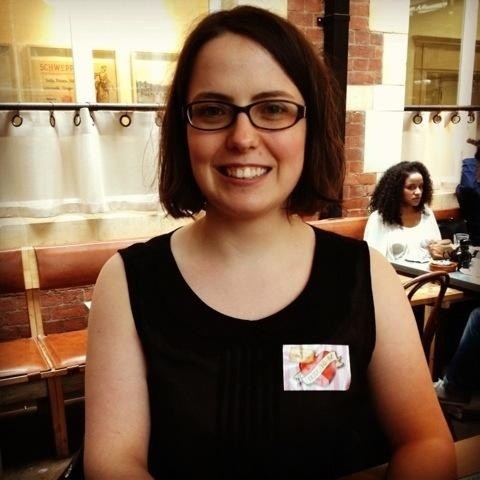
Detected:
[433,207,464,237]
[0,245,66,464]
[398,269,451,361]
[27,239,153,459]
[307,215,370,240]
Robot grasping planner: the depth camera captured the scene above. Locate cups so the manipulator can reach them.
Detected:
[453,233,471,247]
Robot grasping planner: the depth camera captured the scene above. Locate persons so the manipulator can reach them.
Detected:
[83,6,458,480]
[432,309,480,404]
[360,161,442,257]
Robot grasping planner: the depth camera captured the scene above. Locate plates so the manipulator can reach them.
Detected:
[429,261,458,272]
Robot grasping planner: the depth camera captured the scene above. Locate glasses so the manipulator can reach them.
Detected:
[181,98,314,131]
